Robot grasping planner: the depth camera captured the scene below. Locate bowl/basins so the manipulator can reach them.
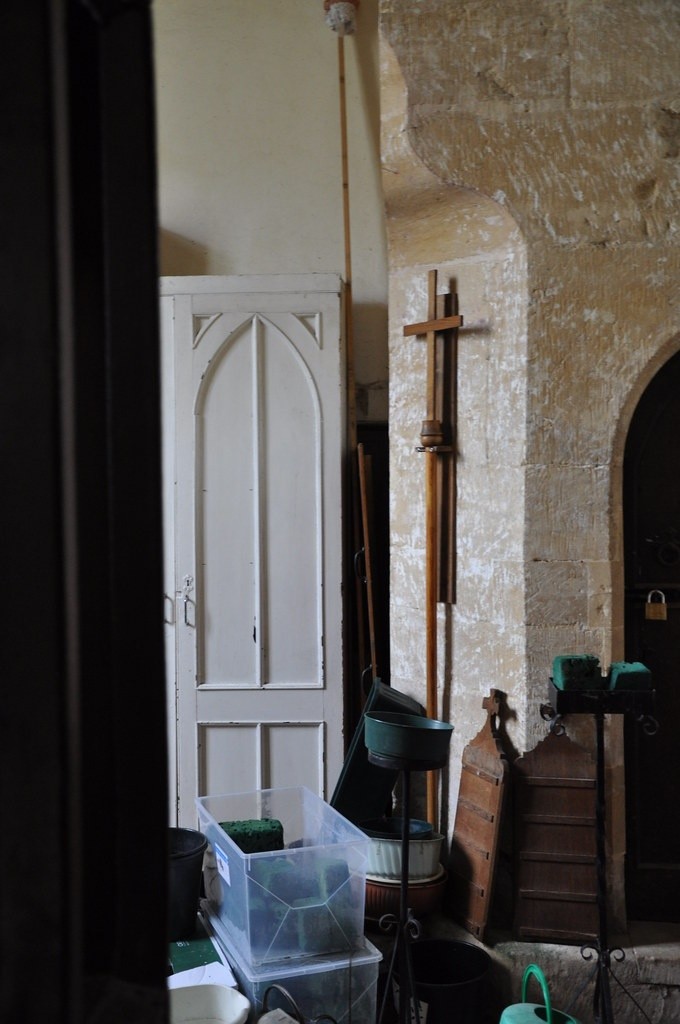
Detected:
[364,710,454,771]
[359,815,447,932]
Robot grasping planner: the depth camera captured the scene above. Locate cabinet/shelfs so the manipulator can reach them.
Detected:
[159,272,347,854]
[365,750,450,1024]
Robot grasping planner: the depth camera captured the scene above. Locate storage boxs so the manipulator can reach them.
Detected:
[329,677,428,830]
[195,784,374,966]
[199,899,383,1024]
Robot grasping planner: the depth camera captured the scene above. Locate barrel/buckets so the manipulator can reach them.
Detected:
[166,825,206,942]
[386,939,492,1024]
[169,985,251,1024]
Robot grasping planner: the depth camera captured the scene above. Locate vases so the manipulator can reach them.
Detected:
[168,985,251,1024]
[364,711,454,761]
[361,818,444,923]
[388,937,493,1024]
[169,827,208,926]
